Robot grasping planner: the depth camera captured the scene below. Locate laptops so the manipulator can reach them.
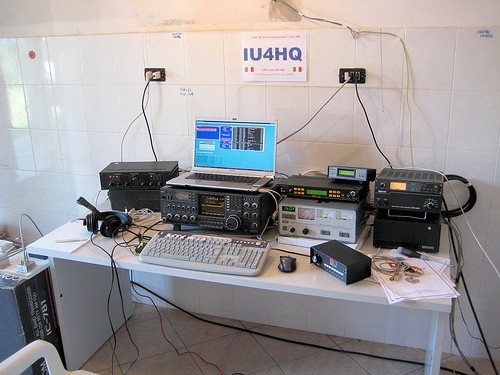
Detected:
[165,120,277,191]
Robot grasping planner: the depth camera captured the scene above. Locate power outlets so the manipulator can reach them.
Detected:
[339,68,366,84]
[144,67,165,82]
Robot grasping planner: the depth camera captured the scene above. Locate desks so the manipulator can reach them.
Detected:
[27,220,459,375]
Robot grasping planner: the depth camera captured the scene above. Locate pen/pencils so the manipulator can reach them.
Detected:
[56,238,87,243]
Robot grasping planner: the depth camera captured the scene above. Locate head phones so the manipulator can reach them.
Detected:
[83,211,132,238]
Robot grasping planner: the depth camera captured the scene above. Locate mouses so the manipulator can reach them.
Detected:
[278,256,297,273]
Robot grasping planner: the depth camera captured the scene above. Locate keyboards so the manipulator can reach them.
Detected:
[139,230,270,276]
